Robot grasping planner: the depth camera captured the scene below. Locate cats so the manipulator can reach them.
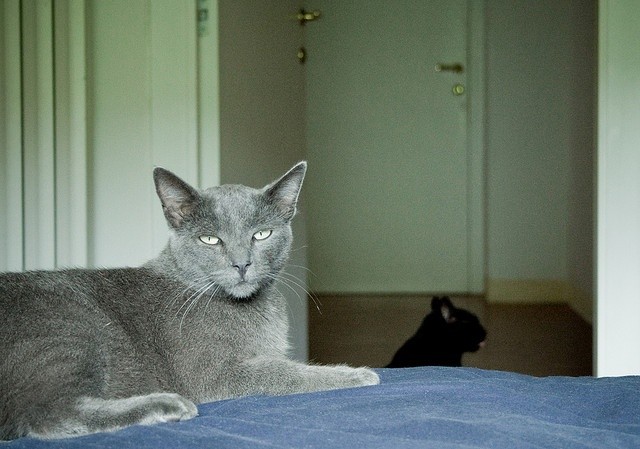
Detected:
[379,294,490,369]
[0,159,382,443]
